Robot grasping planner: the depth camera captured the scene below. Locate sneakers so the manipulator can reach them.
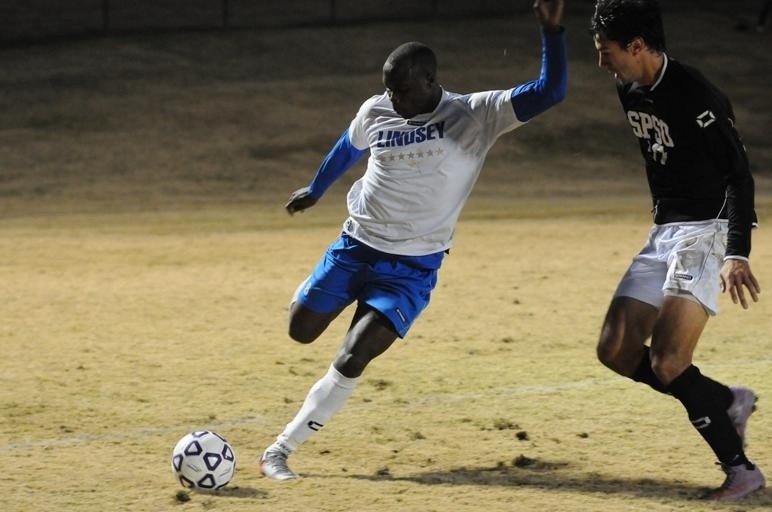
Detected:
[727,387,758,448]
[704,464,764,502]
[261,452,297,480]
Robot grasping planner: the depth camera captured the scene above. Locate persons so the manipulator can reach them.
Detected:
[584,1,765,502]
[259,0,568,481]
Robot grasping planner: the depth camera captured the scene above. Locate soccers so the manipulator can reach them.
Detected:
[172,430,235,493]
